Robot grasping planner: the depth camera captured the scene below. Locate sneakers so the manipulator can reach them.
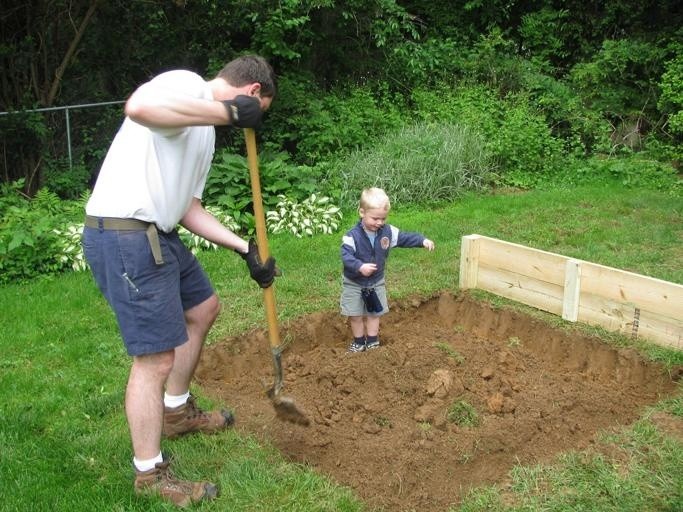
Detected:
[159,391,235,444]
[347,340,380,354]
[128,460,220,512]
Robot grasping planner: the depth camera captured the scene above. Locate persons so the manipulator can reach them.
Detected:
[75,54,277,508]
[337,185,434,354]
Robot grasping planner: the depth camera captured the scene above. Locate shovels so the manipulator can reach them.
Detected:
[243,127,311,427]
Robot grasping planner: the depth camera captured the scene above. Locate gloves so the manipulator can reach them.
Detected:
[233,237,282,289]
[218,93,265,129]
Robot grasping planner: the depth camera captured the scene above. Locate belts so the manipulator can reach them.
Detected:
[82,211,167,267]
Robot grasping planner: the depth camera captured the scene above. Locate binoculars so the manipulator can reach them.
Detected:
[361,287,383,313]
